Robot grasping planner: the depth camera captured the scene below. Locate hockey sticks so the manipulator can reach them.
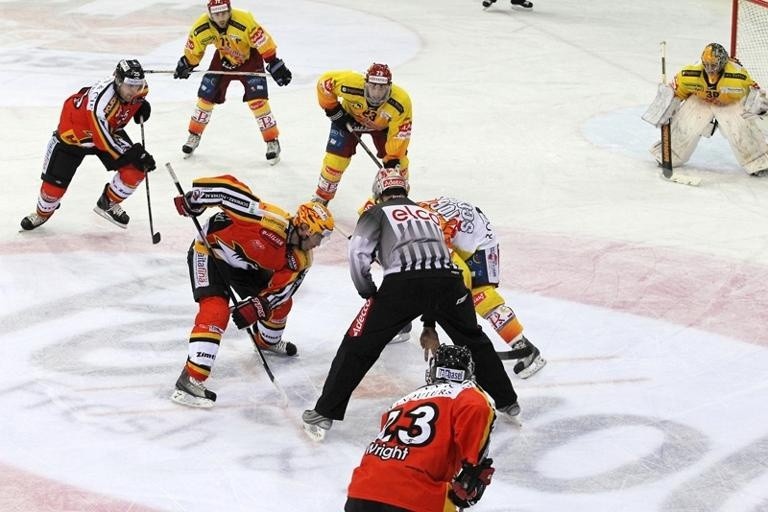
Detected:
[166,162,289,408]
[140,115,161,244]
[658,41,703,186]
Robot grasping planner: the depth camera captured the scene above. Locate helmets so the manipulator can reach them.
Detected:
[207,0,231,21]
[372,167,409,205]
[700,43,728,76]
[363,62,391,106]
[114,59,145,101]
[425,343,475,386]
[292,202,333,249]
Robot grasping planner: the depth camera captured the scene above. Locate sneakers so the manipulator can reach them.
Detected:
[510,0,531,7]
[183,133,199,153]
[265,139,279,159]
[482,0,495,6]
[302,409,331,431]
[498,402,519,416]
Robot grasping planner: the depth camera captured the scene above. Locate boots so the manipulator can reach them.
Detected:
[21,203,59,230]
[97,183,129,224]
[310,195,328,207]
[511,335,539,374]
[251,321,295,356]
[175,354,216,402]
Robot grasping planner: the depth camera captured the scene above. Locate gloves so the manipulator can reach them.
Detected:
[228,295,271,329]
[266,58,291,86]
[174,191,206,217]
[383,158,398,168]
[324,104,356,131]
[447,458,493,510]
[173,55,198,79]
[124,142,155,171]
[133,99,150,123]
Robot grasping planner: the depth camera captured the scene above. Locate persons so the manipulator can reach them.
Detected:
[301,167,520,430]
[309,61,412,216]
[174,0,291,160]
[20,59,156,230]
[174,174,335,402]
[648,42,768,176]
[483,0,533,8]
[357,196,540,375]
[344,343,497,512]
